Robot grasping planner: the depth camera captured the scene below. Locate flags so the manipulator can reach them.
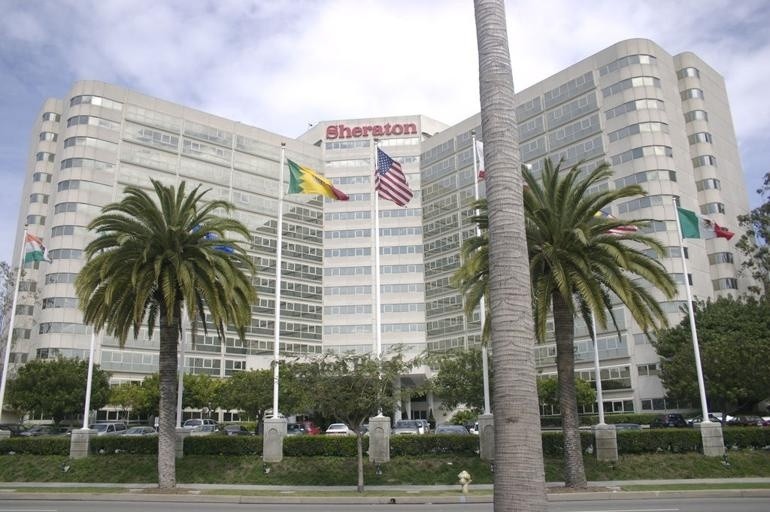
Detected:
[193,225,233,254]
[287,158,349,200]
[593,210,640,234]
[24,233,52,263]
[678,208,735,240]
[375,146,413,206]
[477,140,532,178]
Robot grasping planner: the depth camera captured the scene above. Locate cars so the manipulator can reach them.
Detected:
[287,419,479,434]
[0,419,256,437]
[616,412,769,429]
